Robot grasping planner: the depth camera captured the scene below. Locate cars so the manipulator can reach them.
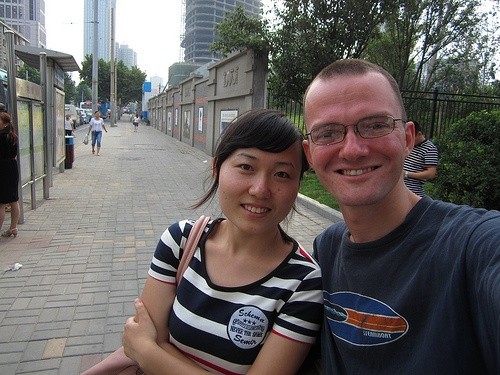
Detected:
[65,104,93,133]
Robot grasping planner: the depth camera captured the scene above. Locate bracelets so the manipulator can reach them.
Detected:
[404,171,408,177]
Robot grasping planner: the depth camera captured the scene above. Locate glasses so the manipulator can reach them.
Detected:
[310,115,407,145]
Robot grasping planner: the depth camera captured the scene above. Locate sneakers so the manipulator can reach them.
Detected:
[91,151,101,156]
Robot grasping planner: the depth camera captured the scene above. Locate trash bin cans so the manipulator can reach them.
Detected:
[64,135,75,170]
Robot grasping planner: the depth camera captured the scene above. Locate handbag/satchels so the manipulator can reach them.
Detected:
[82,216,212,375]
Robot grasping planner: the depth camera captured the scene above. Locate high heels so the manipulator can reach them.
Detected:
[1,227,18,238]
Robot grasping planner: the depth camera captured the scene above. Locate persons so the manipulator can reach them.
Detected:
[302,59,500,375]
[403,120,439,197]
[122,109,324,375]
[65,113,74,136]
[0,112,20,240]
[132,114,139,132]
[87,111,107,156]
